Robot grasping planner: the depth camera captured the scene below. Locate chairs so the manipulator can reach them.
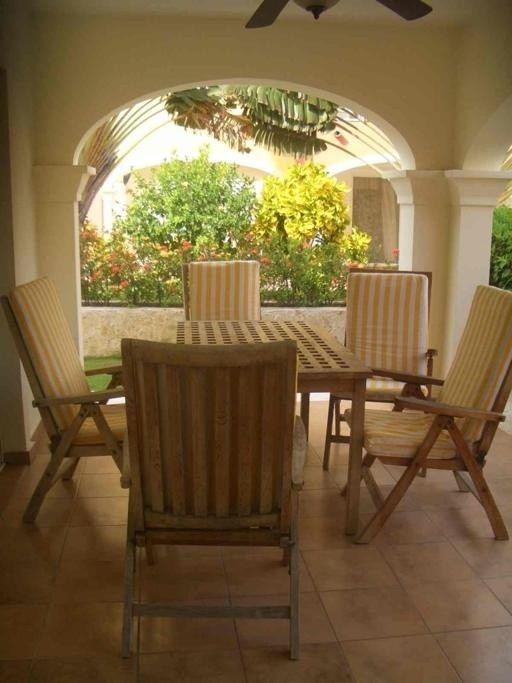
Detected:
[182,260,262,321]
[340,284,510,543]
[325,268,438,477]
[0,273,126,524]
[119,337,307,659]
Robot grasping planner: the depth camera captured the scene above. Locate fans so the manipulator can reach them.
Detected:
[244,0,433,29]
